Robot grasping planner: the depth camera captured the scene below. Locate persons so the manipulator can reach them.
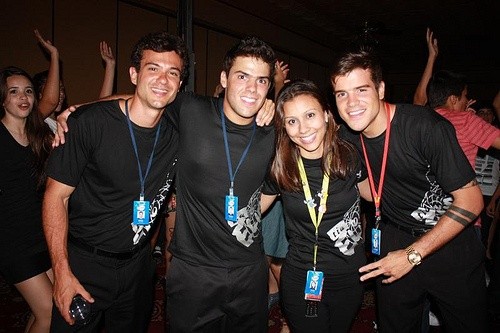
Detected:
[0,66,57,333]
[416,28,500,326]
[334,53,484,333]
[228,207,234,215]
[148,60,289,318]
[31,30,116,118]
[53,37,277,333]
[259,79,375,333]
[373,240,378,249]
[138,212,144,220]
[310,281,316,290]
[40,24,276,332]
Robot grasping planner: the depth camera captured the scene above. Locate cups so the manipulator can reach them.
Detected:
[68,295,94,330]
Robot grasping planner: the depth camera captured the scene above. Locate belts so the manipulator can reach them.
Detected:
[68,233,150,261]
[383,216,433,238]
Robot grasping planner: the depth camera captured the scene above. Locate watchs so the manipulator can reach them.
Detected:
[406,246,422,266]
